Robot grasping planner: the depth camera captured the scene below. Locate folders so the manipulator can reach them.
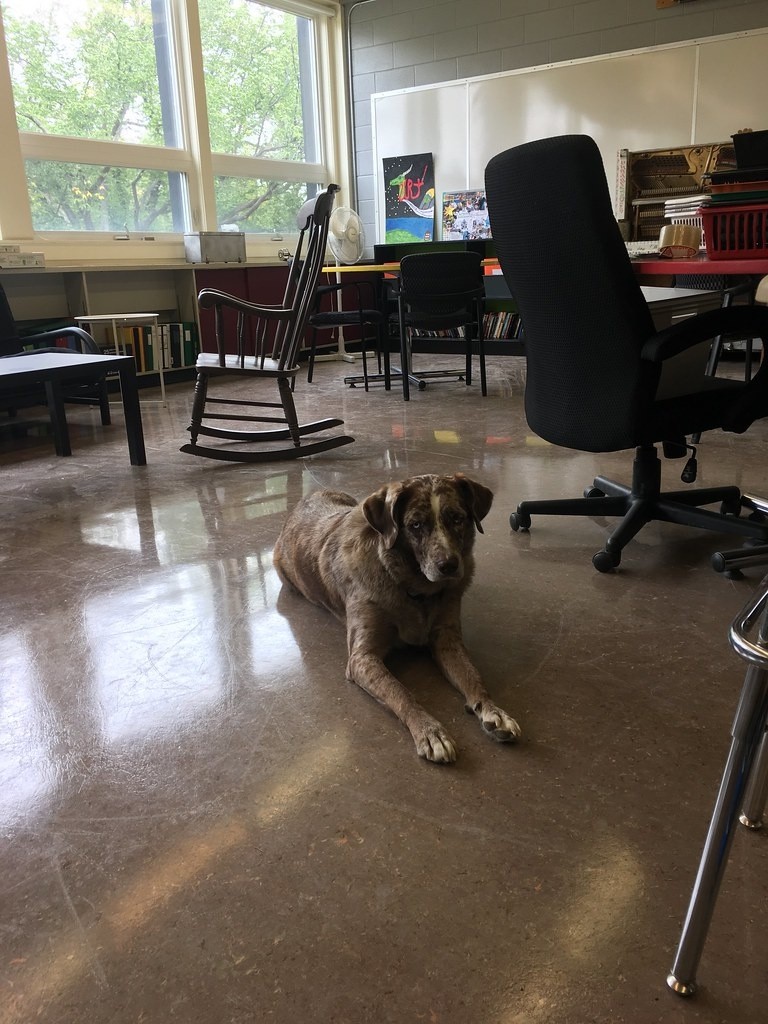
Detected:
[22,322,78,351]
[105,322,201,372]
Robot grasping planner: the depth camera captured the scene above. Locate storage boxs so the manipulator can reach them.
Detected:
[183,230,247,264]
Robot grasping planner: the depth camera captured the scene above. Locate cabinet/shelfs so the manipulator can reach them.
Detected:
[373,238,528,356]
[0,261,378,397]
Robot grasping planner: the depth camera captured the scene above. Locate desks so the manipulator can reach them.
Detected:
[74,314,166,410]
[631,247,768,275]
[0,352,147,467]
[321,261,502,390]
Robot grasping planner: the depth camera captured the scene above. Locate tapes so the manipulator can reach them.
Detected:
[658,222,702,257]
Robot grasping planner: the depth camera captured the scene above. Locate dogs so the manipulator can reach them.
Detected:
[271,469,525,764]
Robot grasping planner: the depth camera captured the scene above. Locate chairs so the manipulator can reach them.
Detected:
[179,183,355,463]
[0,283,111,426]
[286,259,382,392]
[384,251,488,401]
[483,136,768,572]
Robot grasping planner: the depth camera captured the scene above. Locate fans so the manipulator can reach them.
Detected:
[307,205,375,363]
